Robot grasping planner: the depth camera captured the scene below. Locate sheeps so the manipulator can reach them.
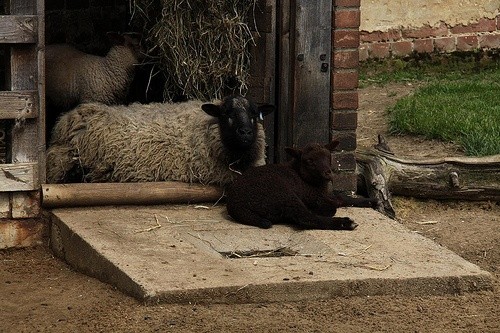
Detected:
[226,142,381,230]
[44,28,145,104]
[46,90,276,194]
